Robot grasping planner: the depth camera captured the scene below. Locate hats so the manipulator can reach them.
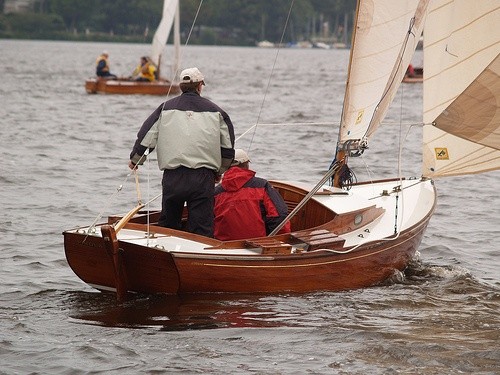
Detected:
[180,67,205,86]
[233,148,254,164]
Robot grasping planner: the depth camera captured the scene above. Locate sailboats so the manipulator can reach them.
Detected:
[59,0,500,298]
[83,0,182,96]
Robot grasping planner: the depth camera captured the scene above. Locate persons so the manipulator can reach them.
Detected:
[406,64,416,78]
[95,52,161,82]
[128,68,236,237]
[213,148,291,242]
